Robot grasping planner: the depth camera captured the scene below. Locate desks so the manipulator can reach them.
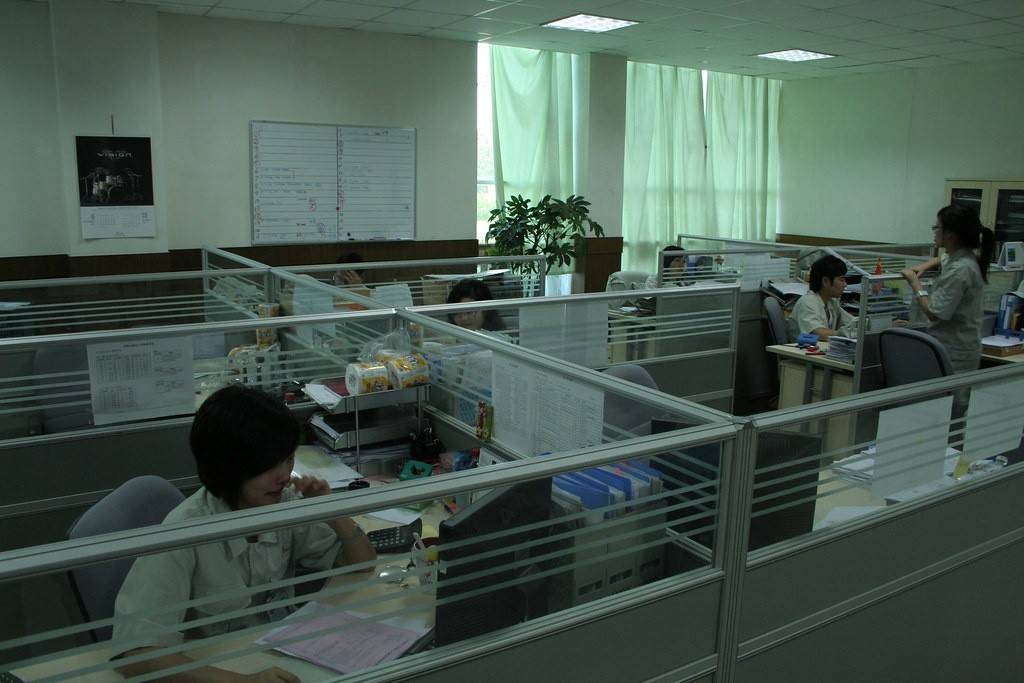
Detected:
[606,309,656,363]
[764,340,857,468]
[811,470,885,531]
[979,353,1024,363]
[0,477,569,683]
[195,357,231,415]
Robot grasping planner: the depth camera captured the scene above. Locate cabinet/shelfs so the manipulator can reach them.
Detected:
[938,178,1024,264]
[300,377,430,477]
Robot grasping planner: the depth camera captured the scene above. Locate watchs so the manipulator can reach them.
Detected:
[916,290,929,298]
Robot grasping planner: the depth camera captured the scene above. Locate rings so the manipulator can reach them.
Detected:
[351,277,353,278]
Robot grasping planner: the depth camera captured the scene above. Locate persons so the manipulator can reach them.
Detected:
[900,205,995,372]
[788,255,908,344]
[642,246,687,291]
[107,385,377,682]
[447,277,511,343]
[320,252,371,307]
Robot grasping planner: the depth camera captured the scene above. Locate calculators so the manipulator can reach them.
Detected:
[366,517,423,556]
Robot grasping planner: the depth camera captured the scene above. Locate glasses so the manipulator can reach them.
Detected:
[333,274,349,283]
[932,225,949,231]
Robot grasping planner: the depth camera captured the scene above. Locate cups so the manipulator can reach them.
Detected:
[414,536,440,594]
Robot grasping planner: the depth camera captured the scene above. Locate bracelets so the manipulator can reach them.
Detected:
[344,527,363,545]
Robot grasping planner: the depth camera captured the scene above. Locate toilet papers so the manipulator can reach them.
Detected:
[258,303,280,318]
[227,347,244,367]
[344,361,388,395]
[388,352,430,389]
[256,328,279,345]
[375,348,393,366]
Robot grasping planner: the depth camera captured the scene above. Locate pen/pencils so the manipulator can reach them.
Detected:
[413,533,425,550]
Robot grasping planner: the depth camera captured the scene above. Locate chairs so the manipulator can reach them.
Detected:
[764,297,790,405]
[29,347,94,436]
[606,271,649,310]
[61,475,187,642]
[602,365,660,445]
[879,329,969,429]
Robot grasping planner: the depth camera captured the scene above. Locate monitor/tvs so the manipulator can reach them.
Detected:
[434,452,552,649]
[695,257,713,281]
[649,417,822,577]
